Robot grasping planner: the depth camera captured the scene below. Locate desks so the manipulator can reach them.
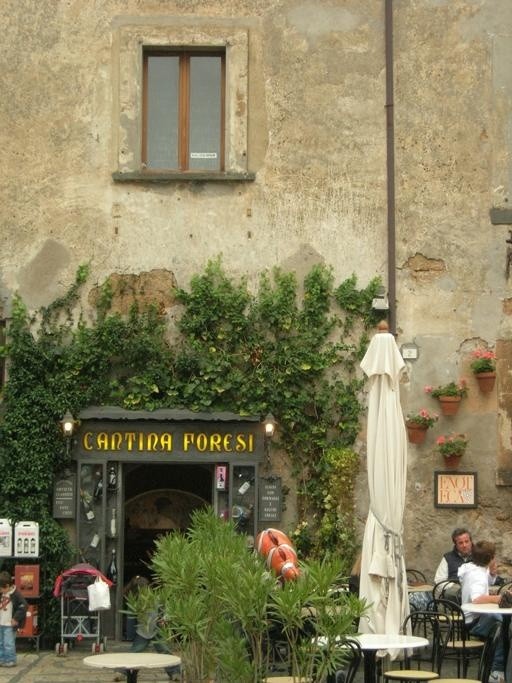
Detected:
[82,652,182,683]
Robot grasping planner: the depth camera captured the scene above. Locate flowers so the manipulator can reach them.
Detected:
[406,408,440,429]
[435,426,471,458]
[468,345,497,373]
[423,376,469,400]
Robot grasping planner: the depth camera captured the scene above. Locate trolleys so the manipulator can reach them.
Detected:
[55,561,115,655]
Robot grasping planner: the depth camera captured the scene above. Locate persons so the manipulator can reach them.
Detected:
[434,526,502,626]
[123,572,151,640]
[113,569,186,682]
[1,571,29,666]
[457,539,508,683]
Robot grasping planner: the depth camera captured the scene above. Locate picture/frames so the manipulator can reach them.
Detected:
[434,471,478,509]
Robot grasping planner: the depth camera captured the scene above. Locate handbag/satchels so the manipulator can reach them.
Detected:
[171,672,181,681]
[87,575,111,612]
[114,673,127,682]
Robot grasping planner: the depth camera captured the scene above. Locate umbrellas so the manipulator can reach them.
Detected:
[356,317,415,673]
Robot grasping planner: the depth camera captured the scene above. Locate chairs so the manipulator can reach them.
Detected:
[227,569,512,683]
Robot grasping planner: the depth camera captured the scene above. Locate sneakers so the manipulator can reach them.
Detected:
[488,670,505,683]
[0,661,16,667]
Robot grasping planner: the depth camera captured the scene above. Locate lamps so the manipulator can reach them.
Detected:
[58,408,81,468]
[261,411,276,470]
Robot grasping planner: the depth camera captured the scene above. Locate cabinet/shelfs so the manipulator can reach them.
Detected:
[0,551,48,655]
[212,462,259,544]
[76,459,125,640]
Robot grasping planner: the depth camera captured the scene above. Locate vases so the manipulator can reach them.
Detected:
[443,455,462,469]
[406,421,428,444]
[438,395,462,416]
[475,372,496,393]
